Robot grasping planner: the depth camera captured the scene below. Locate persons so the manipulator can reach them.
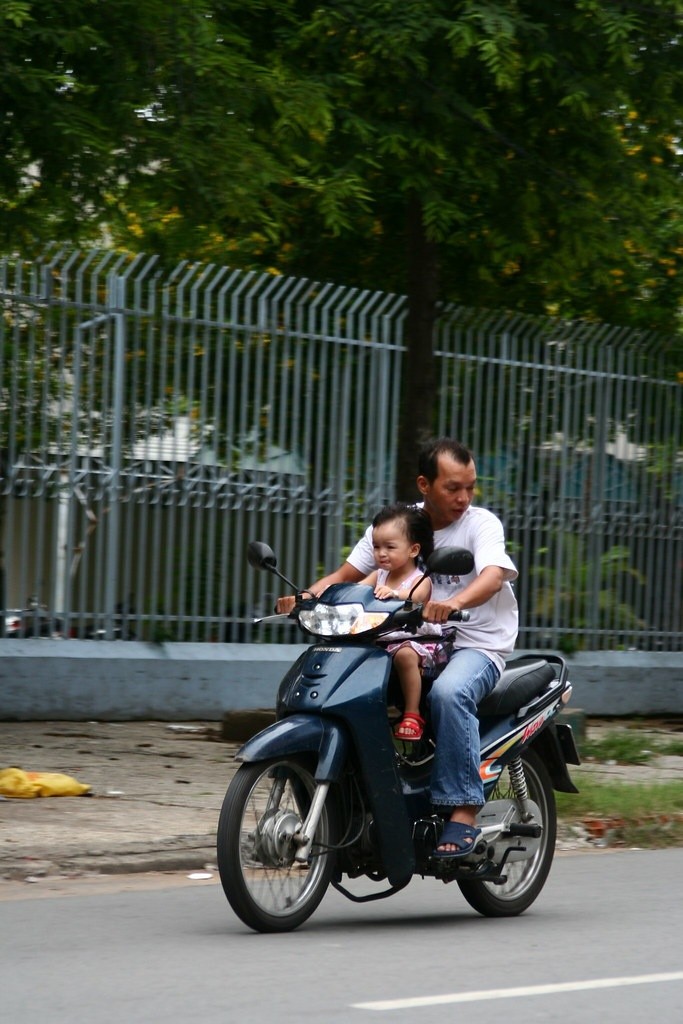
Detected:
[315,503,448,741]
[275,439,519,857]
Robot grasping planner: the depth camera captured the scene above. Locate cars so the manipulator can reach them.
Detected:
[0,607,74,638]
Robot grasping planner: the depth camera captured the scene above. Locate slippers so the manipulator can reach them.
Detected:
[433,820,483,858]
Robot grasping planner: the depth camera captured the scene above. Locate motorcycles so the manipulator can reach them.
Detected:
[211,539,585,934]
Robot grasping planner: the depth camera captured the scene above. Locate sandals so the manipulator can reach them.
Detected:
[396,712,425,741]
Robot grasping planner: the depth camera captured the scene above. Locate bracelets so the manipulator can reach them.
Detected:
[300,589,316,598]
[391,589,399,600]
